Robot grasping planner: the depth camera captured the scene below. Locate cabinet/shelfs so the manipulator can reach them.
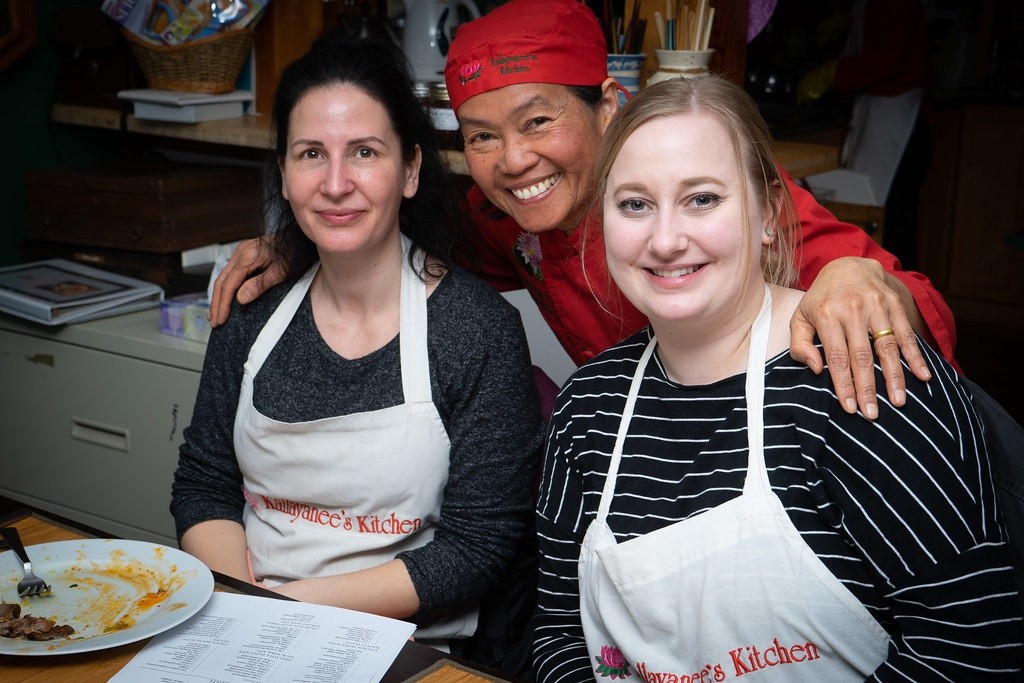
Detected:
[1,0,886,360]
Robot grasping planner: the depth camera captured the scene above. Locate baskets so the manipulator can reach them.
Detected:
[124,27,258,94]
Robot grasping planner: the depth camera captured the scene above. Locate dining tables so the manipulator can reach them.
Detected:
[0,514,509,683]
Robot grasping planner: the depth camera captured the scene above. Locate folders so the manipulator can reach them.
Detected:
[0,258,165,330]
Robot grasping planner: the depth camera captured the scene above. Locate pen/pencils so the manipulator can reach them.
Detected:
[655,0,714,52]
[583,0,648,56]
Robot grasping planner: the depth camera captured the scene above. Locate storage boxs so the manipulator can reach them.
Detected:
[0,303,216,546]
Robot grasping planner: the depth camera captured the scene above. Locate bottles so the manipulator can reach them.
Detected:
[606,53,647,109]
[645,49,714,86]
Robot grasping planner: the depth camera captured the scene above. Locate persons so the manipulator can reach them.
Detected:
[166,39,530,669]
[521,74,1024,683]
[207,0,964,419]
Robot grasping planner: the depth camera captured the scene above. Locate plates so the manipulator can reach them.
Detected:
[1,539,214,656]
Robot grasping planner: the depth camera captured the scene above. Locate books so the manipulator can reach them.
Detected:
[0,258,164,328]
[129,101,243,124]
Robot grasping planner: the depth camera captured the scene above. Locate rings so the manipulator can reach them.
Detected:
[872,329,895,339]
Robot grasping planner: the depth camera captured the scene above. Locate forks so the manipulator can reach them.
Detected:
[0,527,51,597]
